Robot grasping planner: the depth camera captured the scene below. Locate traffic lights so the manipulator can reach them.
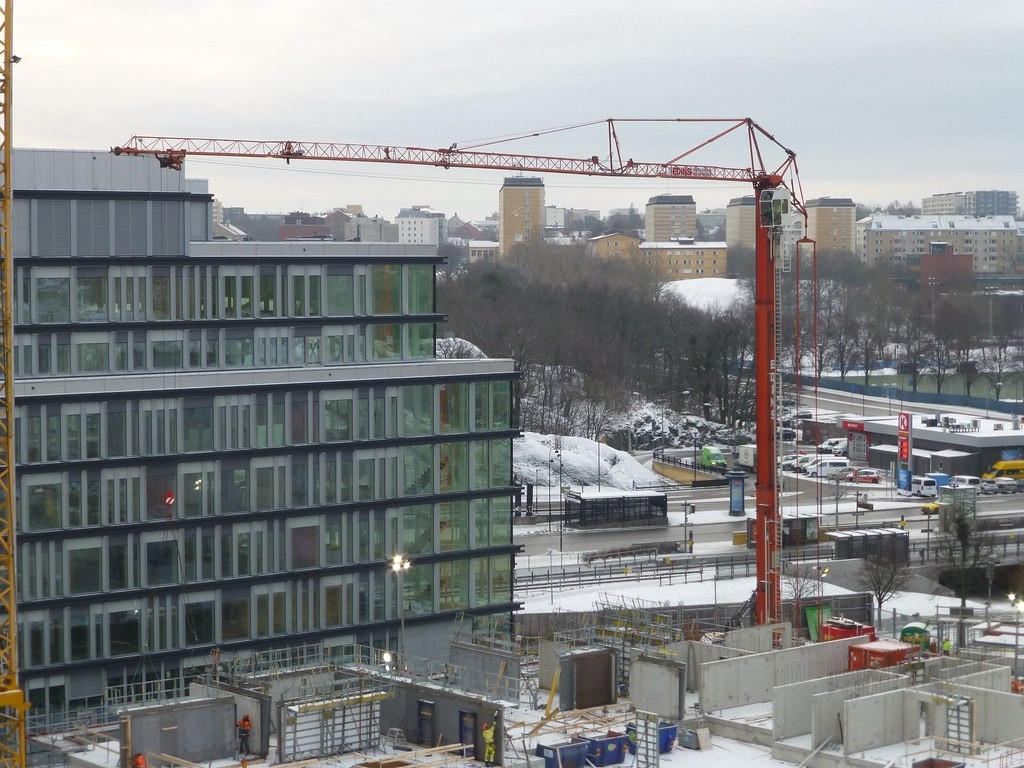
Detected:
[897,439,908,459]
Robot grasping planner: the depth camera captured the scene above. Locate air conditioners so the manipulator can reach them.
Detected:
[995,424,1003,429]
[972,419,981,427]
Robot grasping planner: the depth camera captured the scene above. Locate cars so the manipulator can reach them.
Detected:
[775,409,1024,498]
[922,497,973,514]
[678,457,694,466]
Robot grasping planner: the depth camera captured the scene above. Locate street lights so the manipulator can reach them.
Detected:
[985,286,1000,398]
[548,448,564,552]
[702,402,730,448]
[928,276,941,390]
[632,391,690,462]
[1007,592,1020,694]
[812,566,829,641]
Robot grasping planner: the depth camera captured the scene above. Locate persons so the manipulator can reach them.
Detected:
[943,636,951,655]
[130,753,146,768]
[482,716,497,767]
[236,713,252,755]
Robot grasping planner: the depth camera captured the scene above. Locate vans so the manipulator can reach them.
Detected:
[700,447,727,472]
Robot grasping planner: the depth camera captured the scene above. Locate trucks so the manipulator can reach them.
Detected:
[733,444,757,473]
[847,621,931,672]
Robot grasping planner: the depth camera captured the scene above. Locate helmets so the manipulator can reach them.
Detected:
[243,713,249,721]
[482,722,491,731]
[134,752,141,758]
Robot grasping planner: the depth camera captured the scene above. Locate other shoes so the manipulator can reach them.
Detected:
[238,748,250,755]
[484,760,495,767]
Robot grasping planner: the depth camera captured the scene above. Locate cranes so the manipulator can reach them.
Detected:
[113,118,806,628]
